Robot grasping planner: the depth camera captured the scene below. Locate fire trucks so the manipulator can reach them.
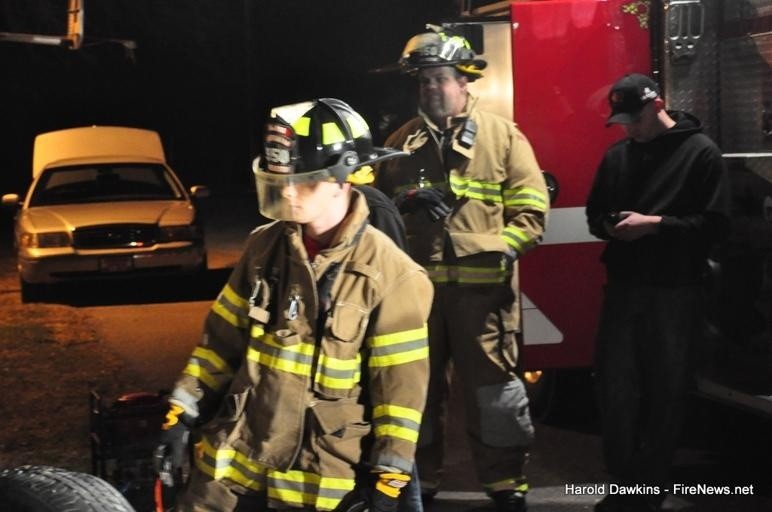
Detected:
[442,1,772,452]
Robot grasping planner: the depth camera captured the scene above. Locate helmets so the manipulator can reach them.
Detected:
[396,24,488,82]
[249,95,410,224]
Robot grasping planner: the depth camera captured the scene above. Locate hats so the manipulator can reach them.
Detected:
[605,73,662,128]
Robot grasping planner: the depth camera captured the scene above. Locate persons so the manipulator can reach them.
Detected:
[585,72,731,512]
[375,32,549,512]
[154,97,435,512]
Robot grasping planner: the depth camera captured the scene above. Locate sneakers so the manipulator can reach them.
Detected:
[492,488,527,511]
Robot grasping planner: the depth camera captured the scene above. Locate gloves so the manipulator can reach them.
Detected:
[365,465,414,511]
[153,398,201,488]
[395,187,452,224]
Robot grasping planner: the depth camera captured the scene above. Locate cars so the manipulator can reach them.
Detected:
[1,121,220,301]
